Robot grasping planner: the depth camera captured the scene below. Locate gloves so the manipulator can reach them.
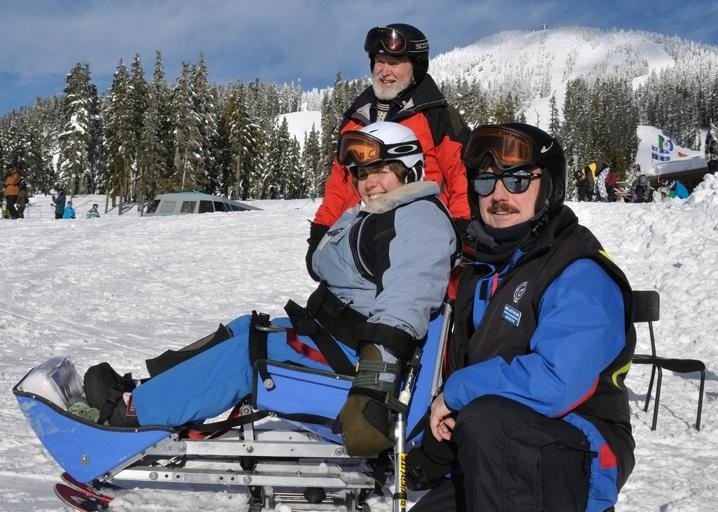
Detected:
[306,223,331,282]
[332,344,401,459]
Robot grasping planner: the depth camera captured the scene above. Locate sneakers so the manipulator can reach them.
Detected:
[83,362,136,427]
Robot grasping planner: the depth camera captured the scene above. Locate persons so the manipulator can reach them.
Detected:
[82,121,457,457]
[406,123,635,512]
[573,160,689,203]
[2,164,100,219]
[306,25,473,301]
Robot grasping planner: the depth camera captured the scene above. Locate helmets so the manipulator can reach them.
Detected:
[639,176,648,186]
[574,170,584,180]
[348,121,424,169]
[465,122,566,237]
[363,23,429,57]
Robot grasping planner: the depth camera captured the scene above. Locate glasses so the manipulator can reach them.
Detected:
[364,26,406,53]
[473,170,541,196]
[337,130,384,165]
[461,125,534,170]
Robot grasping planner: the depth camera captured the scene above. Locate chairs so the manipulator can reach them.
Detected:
[631,289,706,432]
